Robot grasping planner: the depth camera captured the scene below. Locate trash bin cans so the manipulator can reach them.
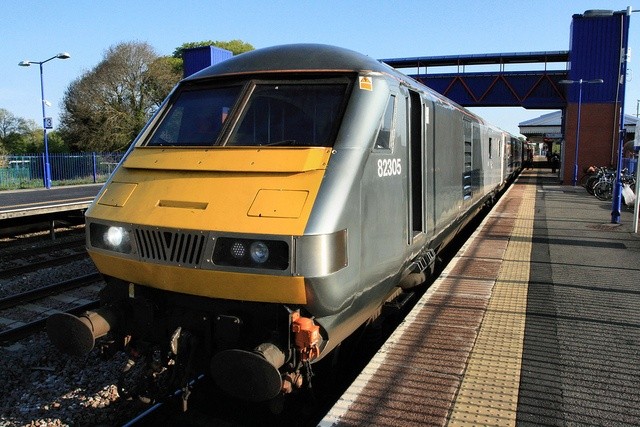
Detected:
[621,157,638,176]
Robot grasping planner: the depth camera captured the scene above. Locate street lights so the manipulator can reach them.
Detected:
[558,78,603,185]
[18,53,69,189]
[583,6,640,225]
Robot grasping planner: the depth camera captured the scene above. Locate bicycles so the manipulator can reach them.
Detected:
[580,164,636,201]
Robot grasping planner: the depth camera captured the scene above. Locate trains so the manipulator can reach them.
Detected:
[47,44,533,403]
